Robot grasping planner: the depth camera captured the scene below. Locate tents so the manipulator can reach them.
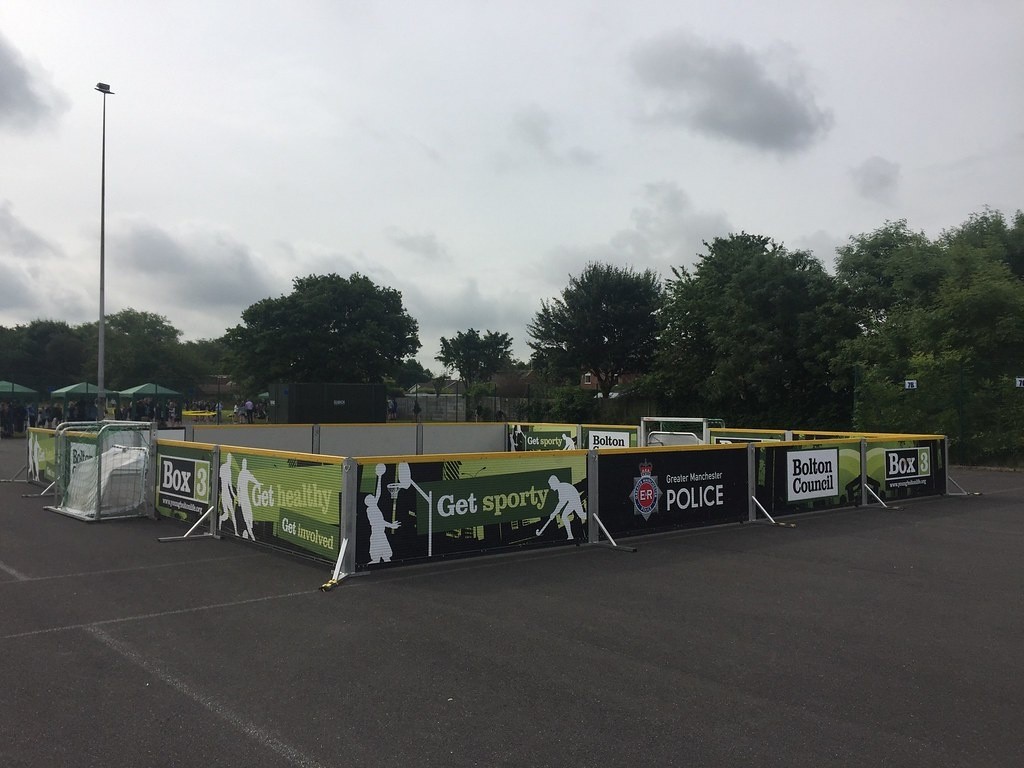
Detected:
[51,382,121,421]
[258,392,270,401]
[117,383,184,421]
[0,380,39,407]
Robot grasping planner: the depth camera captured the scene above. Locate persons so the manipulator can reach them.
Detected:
[387,398,399,420]
[473,402,485,423]
[185,398,225,423]
[495,409,505,422]
[232,398,271,424]
[0,394,184,437]
[411,399,422,423]
